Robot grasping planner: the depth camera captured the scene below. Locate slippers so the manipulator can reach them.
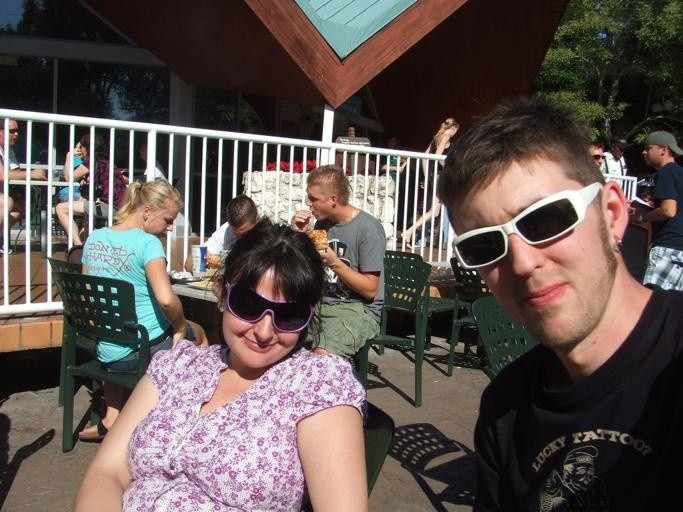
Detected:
[397,236,412,250]
[78,420,109,440]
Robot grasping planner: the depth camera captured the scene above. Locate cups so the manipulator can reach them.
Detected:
[191,245,208,276]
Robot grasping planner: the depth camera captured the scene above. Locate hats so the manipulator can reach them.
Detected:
[612,137,632,149]
[646,131,682,158]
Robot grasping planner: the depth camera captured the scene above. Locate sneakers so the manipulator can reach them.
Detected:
[0,237,12,255]
[415,239,426,247]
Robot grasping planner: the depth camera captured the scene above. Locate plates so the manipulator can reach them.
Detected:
[185,281,215,291]
[169,277,201,284]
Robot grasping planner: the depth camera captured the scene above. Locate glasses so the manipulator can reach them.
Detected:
[1,128,20,134]
[591,154,606,160]
[224,281,317,333]
[451,182,604,272]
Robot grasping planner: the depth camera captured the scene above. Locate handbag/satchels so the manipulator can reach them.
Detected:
[80,182,102,198]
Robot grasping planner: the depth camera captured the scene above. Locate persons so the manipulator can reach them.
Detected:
[55,132,127,250]
[58,140,85,235]
[400,117,460,250]
[436,99,683,512]
[635,129,683,292]
[293,165,386,357]
[74,217,367,511]
[184,196,261,274]
[601,135,628,184]
[589,141,605,172]
[76,179,209,442]
[379,134,406,179]
[137,141,168,182]
[0,117,46,255]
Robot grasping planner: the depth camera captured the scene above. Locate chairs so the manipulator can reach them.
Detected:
[472,295,541,378]
[447,257,492,377]
[302,399,394,511]
[355,251,431,406]
[385,252,471,356]
[53,271,171,452]
[48,257,83,408]
